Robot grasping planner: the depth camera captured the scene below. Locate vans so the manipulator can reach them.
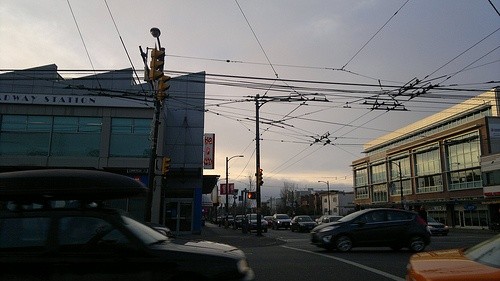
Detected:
[309,208,431,255]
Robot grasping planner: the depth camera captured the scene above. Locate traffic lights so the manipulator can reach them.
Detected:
[161,157,171,177]
[157,75,172,102]
[258,167,264,186]
[149,44,165,82]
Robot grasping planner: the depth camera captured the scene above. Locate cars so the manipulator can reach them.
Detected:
[225,215,234,223]
[404,232,500,281]
[424,215,450,235]
[235,215,246,228]
[264,216,273,227]
[217,216,225,221]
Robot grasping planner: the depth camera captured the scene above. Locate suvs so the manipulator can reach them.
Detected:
[290,215,318,233]
[242,214,268,234]
[0,169,257,281]
[271,213,291,231]
[316,215,344,225]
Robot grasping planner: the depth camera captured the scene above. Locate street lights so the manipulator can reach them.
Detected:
[383,161,404,209]
[318,180,330,215]
[226,155,245,229]
[147,27,166,202]
